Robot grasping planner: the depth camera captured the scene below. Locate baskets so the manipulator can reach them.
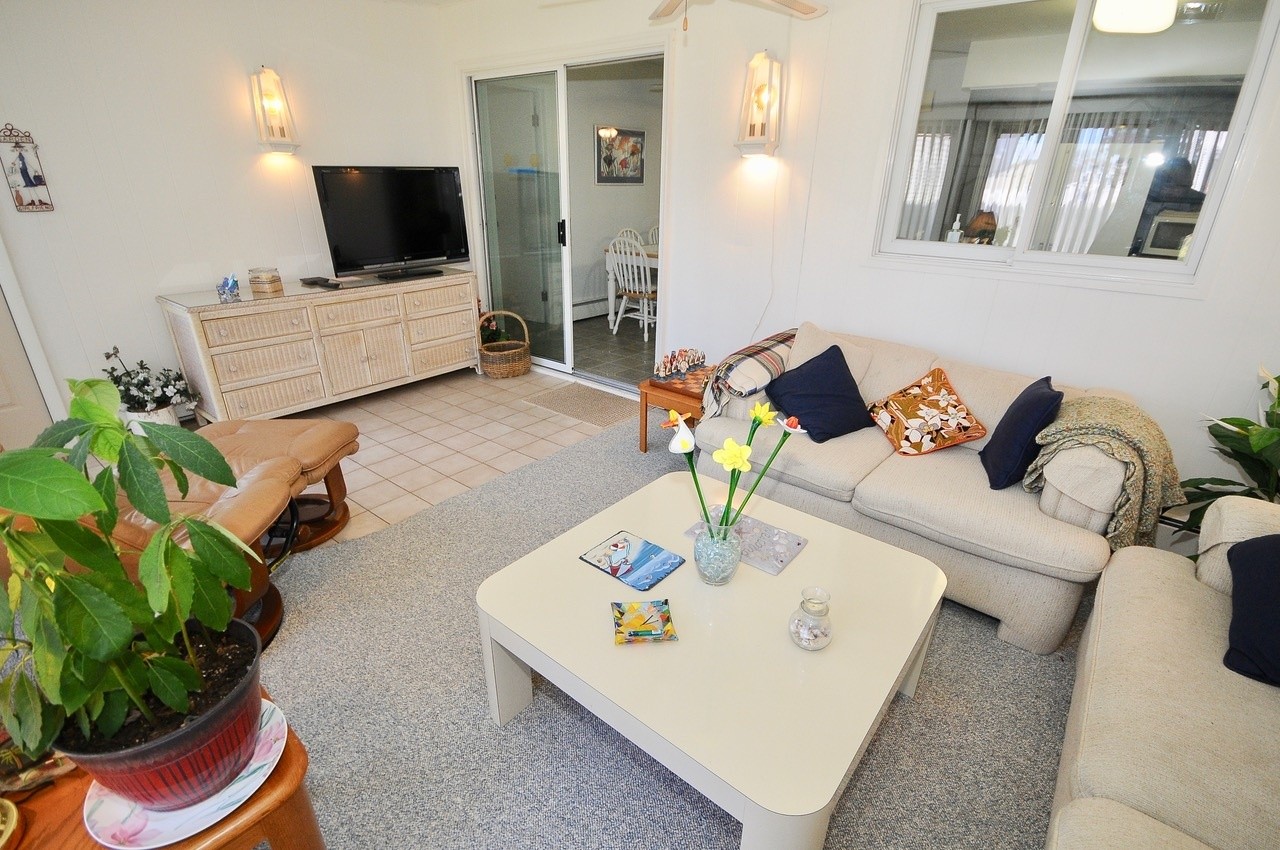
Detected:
[478,311,531,379]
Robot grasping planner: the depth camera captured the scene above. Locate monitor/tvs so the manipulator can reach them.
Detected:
[312,166,470,278]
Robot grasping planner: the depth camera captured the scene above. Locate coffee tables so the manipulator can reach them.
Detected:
[474,472,946,849]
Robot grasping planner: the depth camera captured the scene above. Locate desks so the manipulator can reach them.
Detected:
[604,244,658,330]
[639,379,705,453]
[9,687,329,850]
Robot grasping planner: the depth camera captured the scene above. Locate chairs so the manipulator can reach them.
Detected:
[608,225,660,342]
[3,411,364,649]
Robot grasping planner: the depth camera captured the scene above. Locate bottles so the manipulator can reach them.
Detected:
[788,587,833,650]
[1129,239,1143,256]
[248,267,283,293]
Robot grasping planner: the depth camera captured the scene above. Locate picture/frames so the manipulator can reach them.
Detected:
[593,124,646,185]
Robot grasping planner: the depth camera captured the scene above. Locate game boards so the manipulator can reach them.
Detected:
[648,361,722,399]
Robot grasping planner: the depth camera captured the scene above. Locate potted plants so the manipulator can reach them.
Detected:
[1,363,284,809]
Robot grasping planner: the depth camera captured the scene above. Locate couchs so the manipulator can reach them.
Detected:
[696,322,1128,656]
[1047,496,1279,850]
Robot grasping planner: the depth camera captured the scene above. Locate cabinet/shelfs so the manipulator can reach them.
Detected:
[154,266,486,424]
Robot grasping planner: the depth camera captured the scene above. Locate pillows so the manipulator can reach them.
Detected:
[766,344,1062,488]
[1222,531,1280,685]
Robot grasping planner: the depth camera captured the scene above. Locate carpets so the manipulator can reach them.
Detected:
[523,382,657,429]
[240,409,1094,849]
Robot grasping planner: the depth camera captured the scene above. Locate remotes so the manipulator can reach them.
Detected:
[319,280,341,289]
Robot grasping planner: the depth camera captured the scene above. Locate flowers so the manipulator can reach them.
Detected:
[99,346,201,413]
[661,397,807,539]
[477,298,517,344]
[1159,366,1279,561]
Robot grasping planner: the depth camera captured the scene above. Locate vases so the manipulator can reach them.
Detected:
[123,406,181,436]
[692,504,742,584]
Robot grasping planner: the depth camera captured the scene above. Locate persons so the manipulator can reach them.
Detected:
[654,349,705,382]
[701,375,709,394]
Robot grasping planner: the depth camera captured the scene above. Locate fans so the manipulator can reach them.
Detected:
[649,0,828,23]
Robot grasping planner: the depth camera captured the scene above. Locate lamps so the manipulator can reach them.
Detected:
[252,66,300,148]
[736,51,783,157]
